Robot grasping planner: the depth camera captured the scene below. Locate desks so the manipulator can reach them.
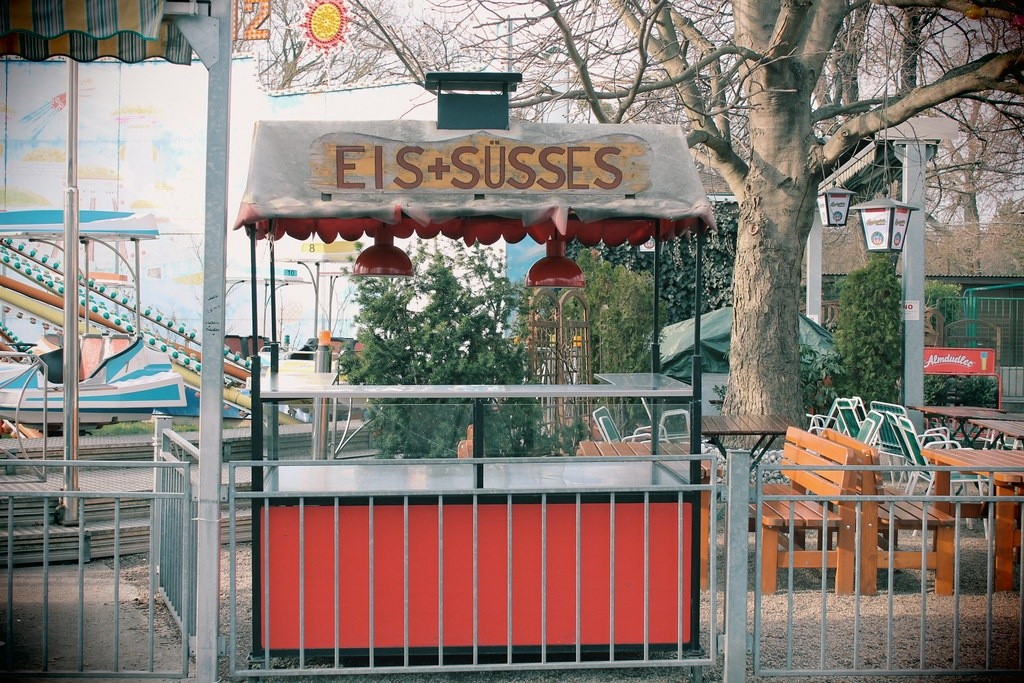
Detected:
[579,441,724,591]
[905,405,1009,446]
[701,415,802,521]
[919,448,1024,592]
[967,419,1024,450]
[916,407,1024,448]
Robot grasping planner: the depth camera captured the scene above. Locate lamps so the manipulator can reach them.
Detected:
[817,181,859,228]
[525,238,586,288]
[846,188,921,254]
[353,236,414,277]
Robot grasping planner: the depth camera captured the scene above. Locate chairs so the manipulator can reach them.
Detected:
[641,397,690,442]
[748,426,857,597]
[806,396,989,541]
[819,427,955,595]
[591,406,670,443]
